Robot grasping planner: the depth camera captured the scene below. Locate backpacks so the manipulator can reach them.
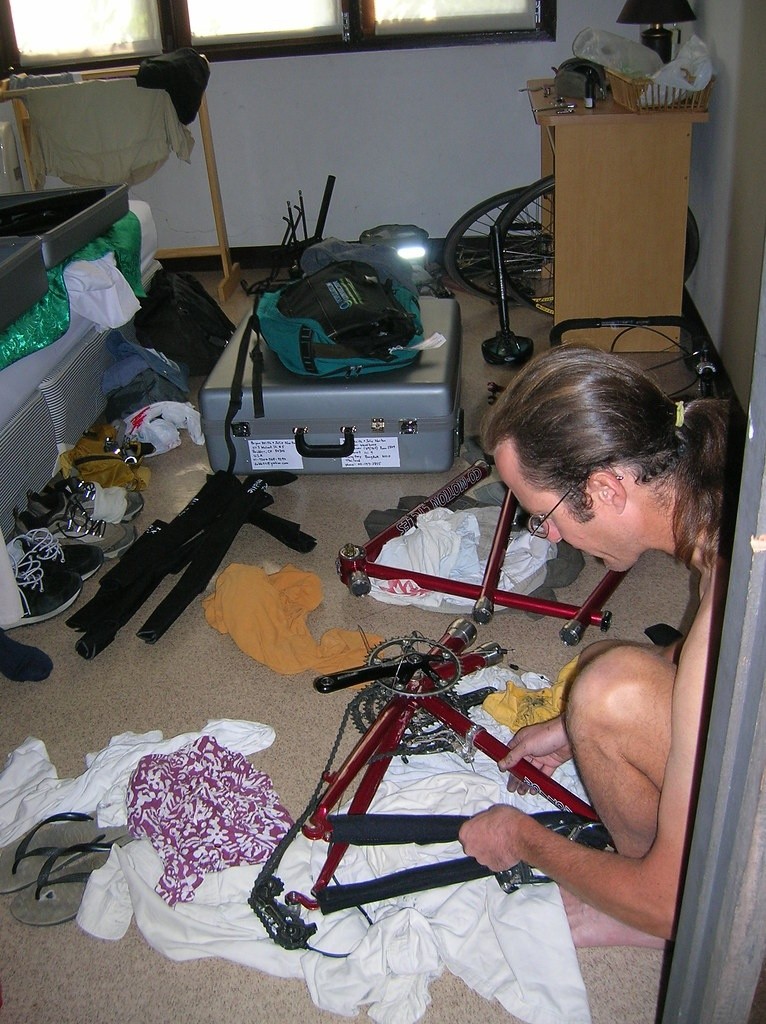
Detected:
[133,268,238,376]
[251,260,424,380]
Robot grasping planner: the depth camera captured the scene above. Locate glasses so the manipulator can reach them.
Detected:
[527,469,624,538]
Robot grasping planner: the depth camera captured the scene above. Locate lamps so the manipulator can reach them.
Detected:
[616,0,697,65]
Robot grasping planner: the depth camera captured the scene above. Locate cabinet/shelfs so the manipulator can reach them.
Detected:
[525,79,710,355]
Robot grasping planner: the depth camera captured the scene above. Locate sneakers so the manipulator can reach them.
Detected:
[1,477,145,633]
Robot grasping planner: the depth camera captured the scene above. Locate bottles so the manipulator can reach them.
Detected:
[585,68,595,109]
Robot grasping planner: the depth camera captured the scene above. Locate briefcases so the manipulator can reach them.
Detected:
[200,290,463,477]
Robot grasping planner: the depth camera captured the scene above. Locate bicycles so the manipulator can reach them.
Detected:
[274,175,706,319]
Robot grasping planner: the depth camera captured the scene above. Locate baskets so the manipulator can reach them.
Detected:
[604,66,715,114]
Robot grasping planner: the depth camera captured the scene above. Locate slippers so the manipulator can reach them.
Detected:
[0,808,147,927]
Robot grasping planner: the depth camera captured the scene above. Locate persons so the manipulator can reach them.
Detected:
[459,345,746,951]
